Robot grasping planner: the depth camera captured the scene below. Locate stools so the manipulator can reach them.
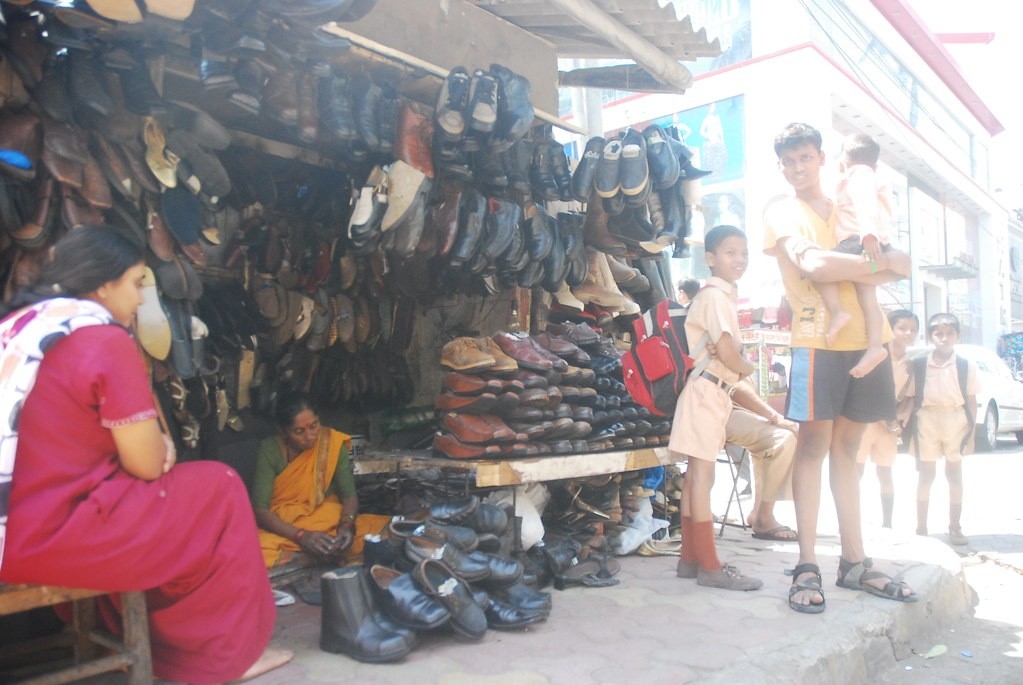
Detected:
[0,573,154,685]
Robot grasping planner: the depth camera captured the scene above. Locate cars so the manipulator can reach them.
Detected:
[904,342,1022,451]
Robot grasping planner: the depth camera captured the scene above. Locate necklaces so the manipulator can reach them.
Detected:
[287,439,290,463]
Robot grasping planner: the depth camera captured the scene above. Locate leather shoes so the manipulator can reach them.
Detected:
[0,0,682,663]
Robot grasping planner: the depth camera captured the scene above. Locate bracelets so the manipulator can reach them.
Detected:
[336,521,356,536]
[871,259,876,273]
[294,528,306,543]
[340,513,354,521]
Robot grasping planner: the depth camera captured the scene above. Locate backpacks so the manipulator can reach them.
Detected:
[621,283,728,418]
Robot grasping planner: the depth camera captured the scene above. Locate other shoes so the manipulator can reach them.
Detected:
[949,523,969,544]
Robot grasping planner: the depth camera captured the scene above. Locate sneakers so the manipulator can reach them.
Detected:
[695,564,763,590]
[676,560,697,578]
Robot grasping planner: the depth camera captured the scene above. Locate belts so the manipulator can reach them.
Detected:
[690,368,735,395]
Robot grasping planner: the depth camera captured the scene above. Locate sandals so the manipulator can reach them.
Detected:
[789,563,826,613]
[836,556,918,601]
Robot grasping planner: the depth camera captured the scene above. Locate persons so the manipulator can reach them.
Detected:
[0,224,294,685]
[811,132,893,379]
[668,225,764,591]
[713,195,741,229]
[669,113,690,144]
[764,123,919,614]
[892,313,977,545]
[700,103,728,180]
[839,309,919,534]
[725,376,799,541]
[677,277,700,307]
[249,391,392,570]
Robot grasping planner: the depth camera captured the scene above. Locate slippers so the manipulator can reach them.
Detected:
[753,526,799,542]
